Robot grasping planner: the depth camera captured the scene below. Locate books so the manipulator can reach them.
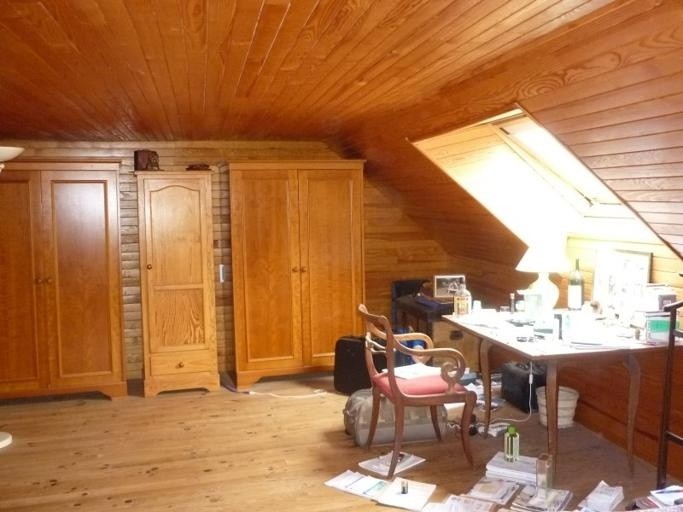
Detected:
[421,451,682,512]
[357,450,427,476]
[323,469,437,511]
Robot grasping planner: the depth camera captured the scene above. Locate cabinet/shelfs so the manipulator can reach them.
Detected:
[224,156,367,390]
[134,169,221,398]
[0,156,127,400]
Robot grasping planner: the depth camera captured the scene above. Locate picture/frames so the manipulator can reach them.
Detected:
[432,274,466,302]
[590,247,653,307]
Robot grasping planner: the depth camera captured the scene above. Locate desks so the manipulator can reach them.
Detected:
[439,308,682,487]
[394,292,480,374]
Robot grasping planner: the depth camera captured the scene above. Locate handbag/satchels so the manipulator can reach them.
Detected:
[501,360,548,413]
[334,336,387,394]
[343,388,448,447]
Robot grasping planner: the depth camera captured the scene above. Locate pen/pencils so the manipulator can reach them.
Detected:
[656,490,683,493]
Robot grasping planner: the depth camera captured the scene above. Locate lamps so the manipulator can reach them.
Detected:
[514,242,572,310]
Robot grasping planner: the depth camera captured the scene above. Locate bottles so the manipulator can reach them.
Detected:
[503,426,519,464]
[454,283,472,317]
[568,259,584,311]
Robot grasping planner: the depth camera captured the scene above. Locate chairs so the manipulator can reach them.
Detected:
[356,303,477,482]
